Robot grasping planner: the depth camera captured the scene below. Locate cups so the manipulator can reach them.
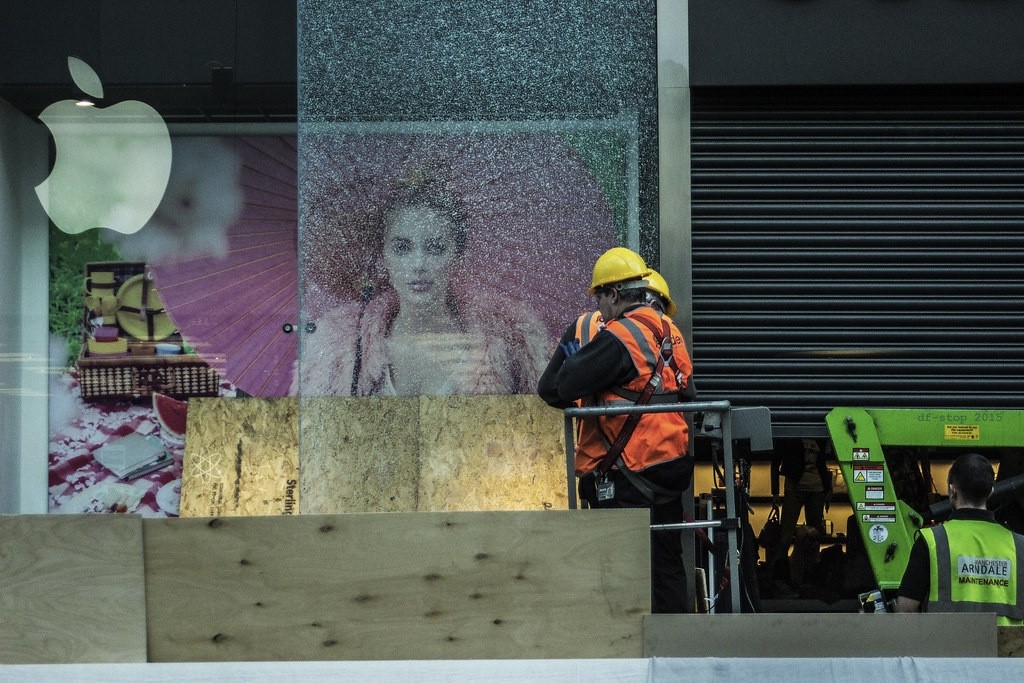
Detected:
[85,272,114,299]
[155,344,181,356]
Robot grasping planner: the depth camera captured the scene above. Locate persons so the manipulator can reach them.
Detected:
[742,524,877,603]
[537,247,696,615]
[895,454,1024,626]
[291,201,562,396]
[770,438,833,554]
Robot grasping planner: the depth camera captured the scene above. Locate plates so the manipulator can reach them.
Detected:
[116,274,176,342]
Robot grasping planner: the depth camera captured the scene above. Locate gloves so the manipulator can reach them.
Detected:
[558,338,580,356]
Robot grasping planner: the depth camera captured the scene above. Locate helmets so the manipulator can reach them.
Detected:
[642,268,677,317]
[587,247,652,297]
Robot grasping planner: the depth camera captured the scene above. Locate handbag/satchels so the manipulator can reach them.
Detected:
[758,506,781,552]
